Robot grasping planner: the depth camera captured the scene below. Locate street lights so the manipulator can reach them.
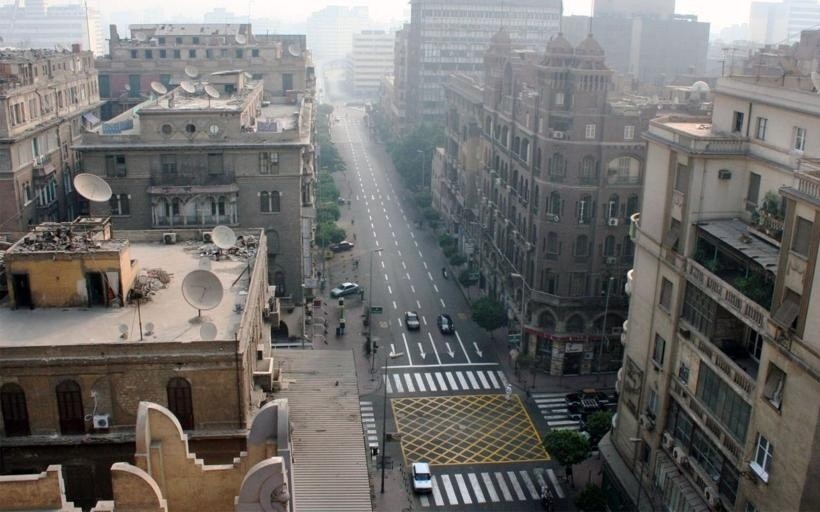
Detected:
[629,437,645,512]
[366,248,384,355]
[511,273,525,354]
[381,352,405,493]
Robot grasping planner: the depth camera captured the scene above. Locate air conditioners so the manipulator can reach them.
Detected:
[661,431,685,465]
[203,230,214,242]
[92,414,110,430]
[162,230,177,245]
[609,218,618,227]
[704,486,718,505]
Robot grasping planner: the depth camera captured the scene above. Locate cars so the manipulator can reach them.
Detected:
[405,311,420,329]
[329,241,354,252]
[330,282,360,298]
[412,462,432,494]
[437,313,455,335]
[564,388,619,430]
